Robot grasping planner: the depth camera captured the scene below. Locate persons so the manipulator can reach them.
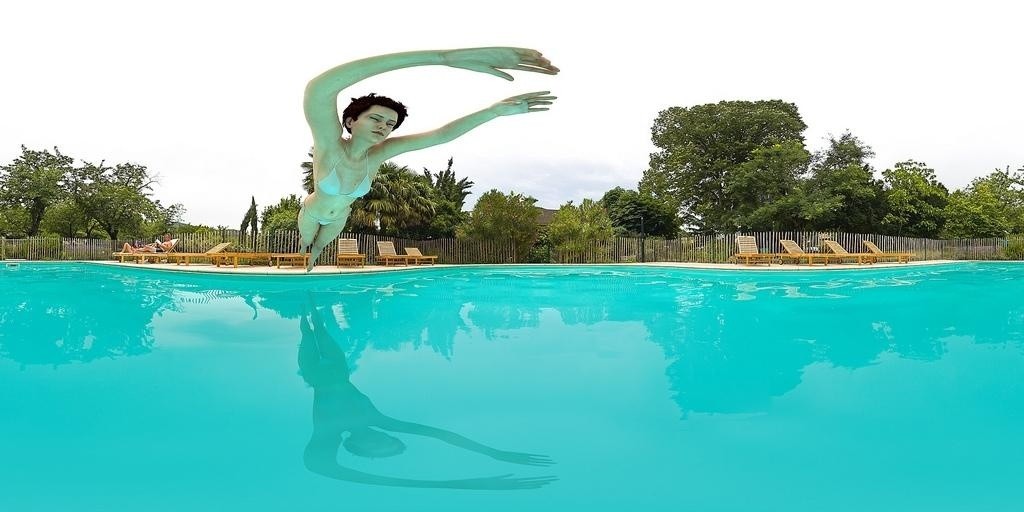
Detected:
[120,233,171,253]
[294,310,556,494]
[288,47,560,272]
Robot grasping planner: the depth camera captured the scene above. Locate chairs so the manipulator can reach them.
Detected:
[337,238,438,268]
[112,239,311,269]
[734,235,917,266]
